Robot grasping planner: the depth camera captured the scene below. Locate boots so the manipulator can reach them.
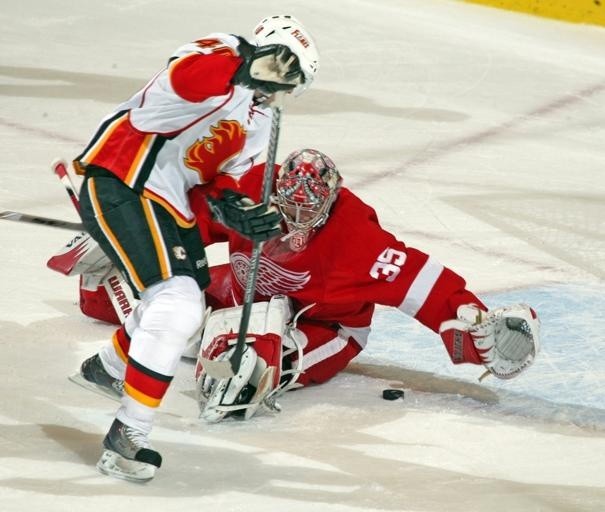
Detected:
[103,418,162,468]
[80,351,128,397]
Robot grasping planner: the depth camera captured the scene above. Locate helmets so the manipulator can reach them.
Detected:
[252,13,322,97]
[276,147,344,212]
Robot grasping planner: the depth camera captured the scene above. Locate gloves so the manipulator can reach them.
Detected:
[240,46,301,97]
[205,189,282,242]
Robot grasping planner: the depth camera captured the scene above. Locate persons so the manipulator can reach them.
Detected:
[49,147,544,424]
[71,12,321,469]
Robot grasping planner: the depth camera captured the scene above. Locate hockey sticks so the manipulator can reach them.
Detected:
[195,89,285,380]
